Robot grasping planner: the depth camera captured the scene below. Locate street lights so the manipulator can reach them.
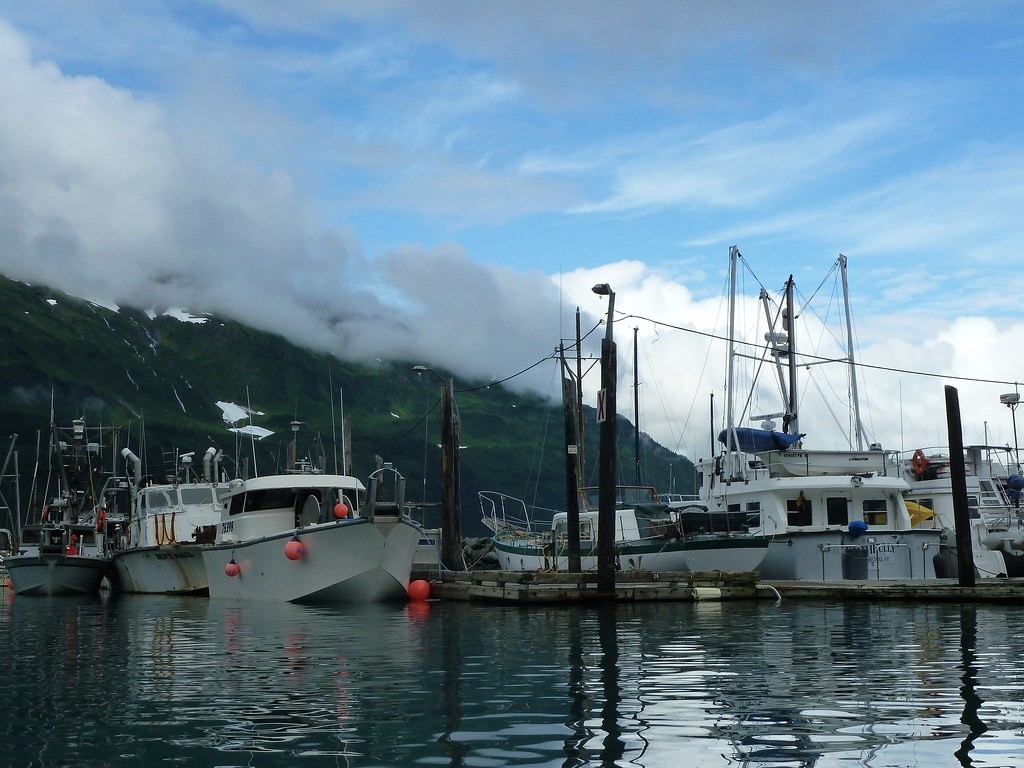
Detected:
[592,283,617,590]
[412,364,459,415]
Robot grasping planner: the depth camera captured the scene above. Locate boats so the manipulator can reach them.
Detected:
[192,384,423,603]
[2,360,443,595]
[476,486,770,572]
[617,245,1024,578]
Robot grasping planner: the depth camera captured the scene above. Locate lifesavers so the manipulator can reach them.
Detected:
[43,506,48,519]
[913,450,926,475]
[97,510,105,532]
